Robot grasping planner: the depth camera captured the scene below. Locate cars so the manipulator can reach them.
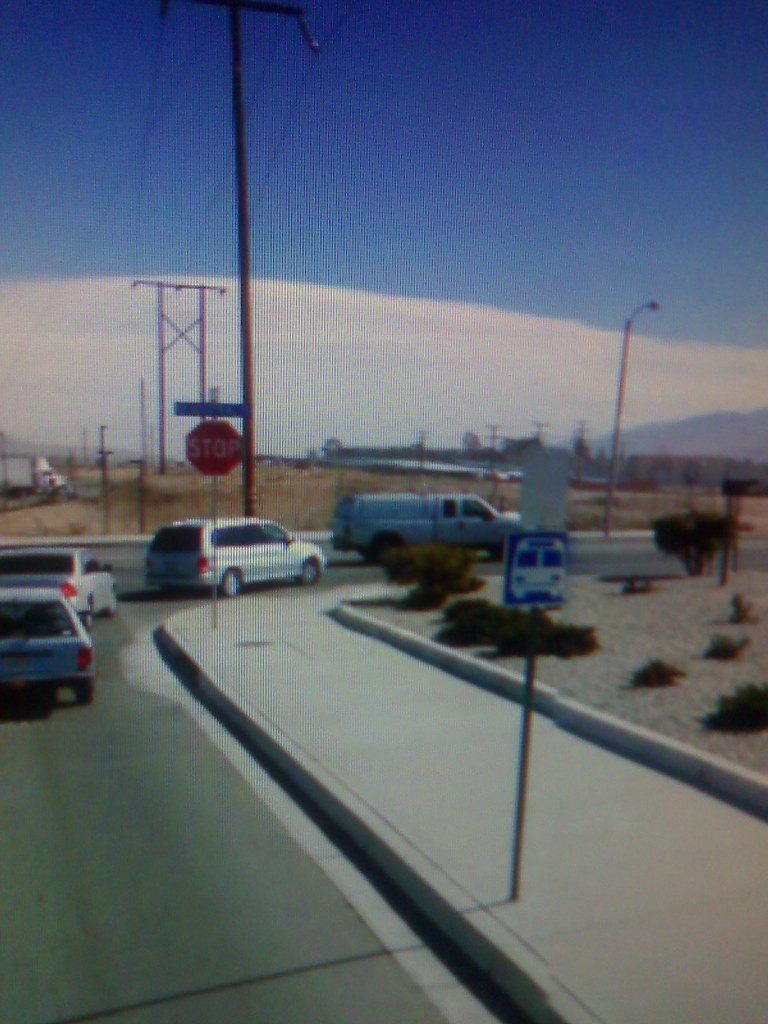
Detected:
[0,544,119,631]
[0,586,98,706]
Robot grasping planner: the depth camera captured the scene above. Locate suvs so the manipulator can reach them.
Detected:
[144,516,328,599]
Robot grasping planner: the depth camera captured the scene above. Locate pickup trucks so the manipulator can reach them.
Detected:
[327,489,523,571]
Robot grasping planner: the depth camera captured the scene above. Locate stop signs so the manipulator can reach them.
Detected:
[185,420,246,479]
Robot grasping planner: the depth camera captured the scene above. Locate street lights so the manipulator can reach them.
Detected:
[129,458,149,535]
[99,423,111,535]
[603,299,664,537]
